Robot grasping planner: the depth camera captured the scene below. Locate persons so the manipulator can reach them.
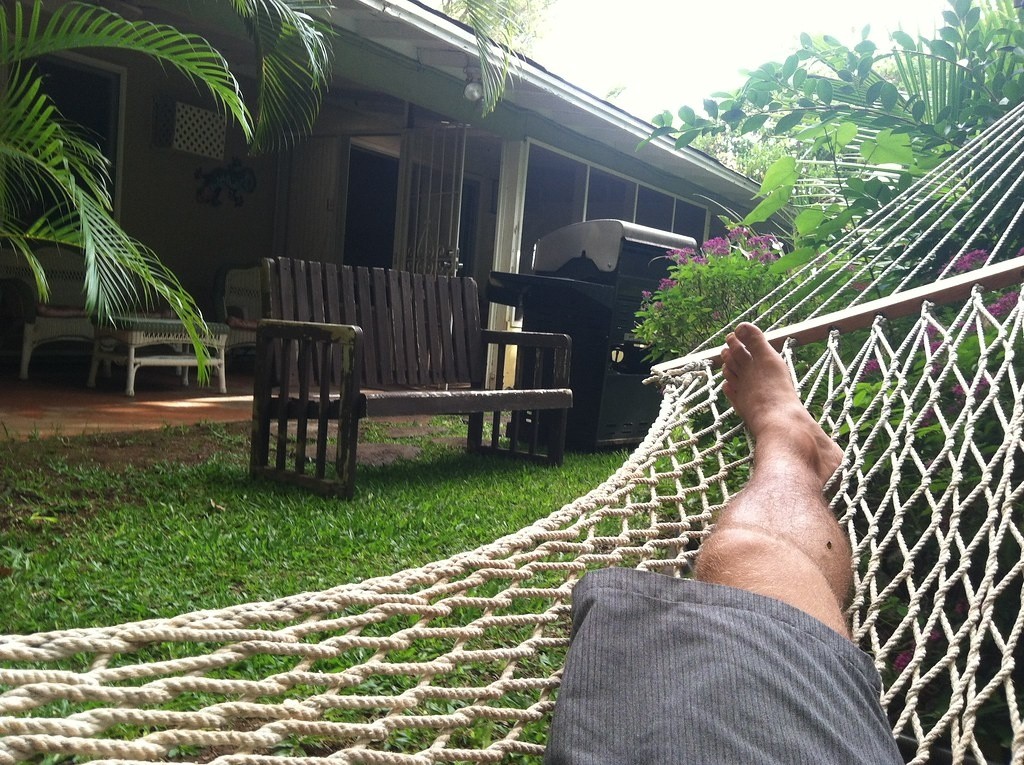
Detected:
[543,322,905,765]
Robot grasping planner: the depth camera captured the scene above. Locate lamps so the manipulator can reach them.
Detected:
[465,55,483,100]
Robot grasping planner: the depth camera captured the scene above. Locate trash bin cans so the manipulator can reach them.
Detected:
[508,218,698,454]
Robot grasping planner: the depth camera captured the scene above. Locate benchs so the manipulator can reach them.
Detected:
[0,245,179,381]
[252,256,573,501]
[87,315,230,397]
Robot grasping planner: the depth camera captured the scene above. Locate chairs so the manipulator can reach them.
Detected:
[213,264,262,378]
